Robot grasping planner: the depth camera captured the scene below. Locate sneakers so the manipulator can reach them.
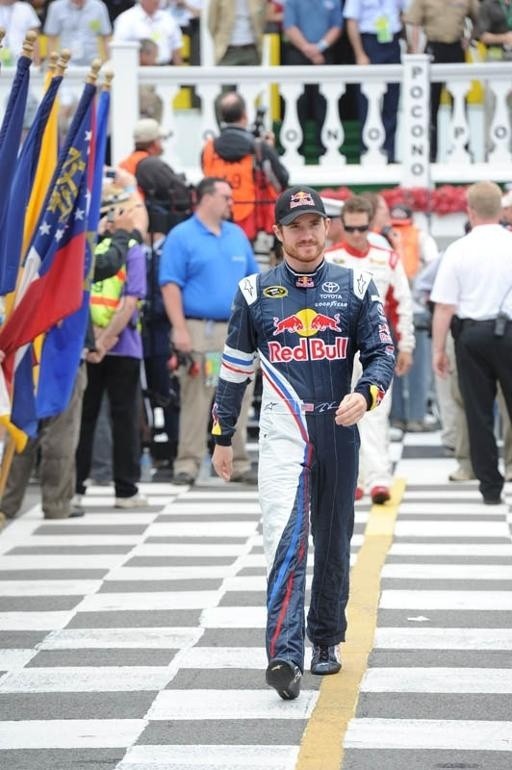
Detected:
[45,505,84,518]
[372,487,389,503]
[265,658,301,700]
[392,421,425,433]
[311,643,341,675]
[450,468,474,480]
[115,492,151,508]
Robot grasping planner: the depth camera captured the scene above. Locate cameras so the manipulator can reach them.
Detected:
[250,105,270,136]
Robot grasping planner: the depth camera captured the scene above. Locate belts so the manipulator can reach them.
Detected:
[462,319,511,328]
[185,315,229,322]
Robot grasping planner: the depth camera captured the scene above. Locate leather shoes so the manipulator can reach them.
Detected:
[174,473,194,484]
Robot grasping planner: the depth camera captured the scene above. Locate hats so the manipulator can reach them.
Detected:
[274,186,328,226]
[133,119,172,143]
[390,206,413,226]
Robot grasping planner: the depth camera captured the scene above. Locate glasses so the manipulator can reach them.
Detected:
[343,222,368,231]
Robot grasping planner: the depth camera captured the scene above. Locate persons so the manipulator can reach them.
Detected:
[211,186,400,703]
[2,2,512,521]
